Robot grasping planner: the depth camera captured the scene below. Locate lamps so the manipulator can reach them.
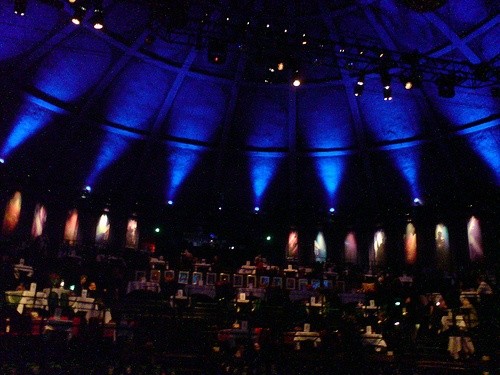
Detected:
[71,5,88,25]
[384,90,392,101]
[354,85,363,96]
[292,69,303,87]
[14,1,26,15]
[93,8,105,29]
[400,76,412,90]
[357,76,364,86]
[383,76,390,88]
